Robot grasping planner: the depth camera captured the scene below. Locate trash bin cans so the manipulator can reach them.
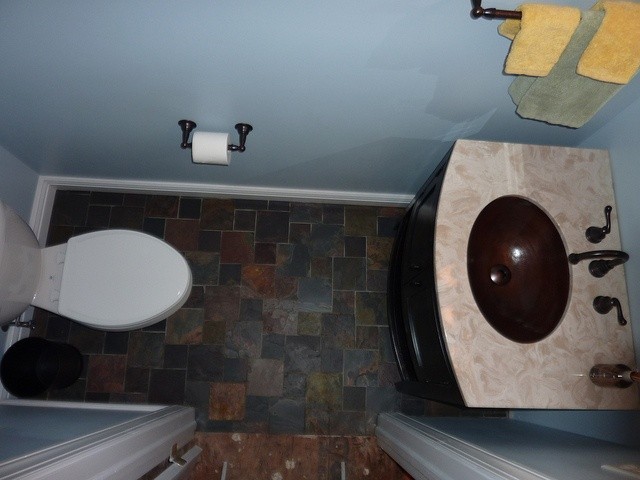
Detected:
[0,335,83,400]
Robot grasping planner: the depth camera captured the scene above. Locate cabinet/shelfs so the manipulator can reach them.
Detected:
[386,138,464,409]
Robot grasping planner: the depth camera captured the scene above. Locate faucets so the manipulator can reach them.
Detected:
[568,250,629,278]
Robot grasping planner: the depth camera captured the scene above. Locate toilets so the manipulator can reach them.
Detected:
[0,201,193,332]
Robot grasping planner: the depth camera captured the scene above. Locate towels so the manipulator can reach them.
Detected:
[576,1,639,85]
[496,2,583,79]
[506,7,639,130]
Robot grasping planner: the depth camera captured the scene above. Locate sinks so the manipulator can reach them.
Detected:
[467,194,573,345]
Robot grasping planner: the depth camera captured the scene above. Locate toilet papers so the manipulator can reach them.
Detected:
[192,131,231,165]
